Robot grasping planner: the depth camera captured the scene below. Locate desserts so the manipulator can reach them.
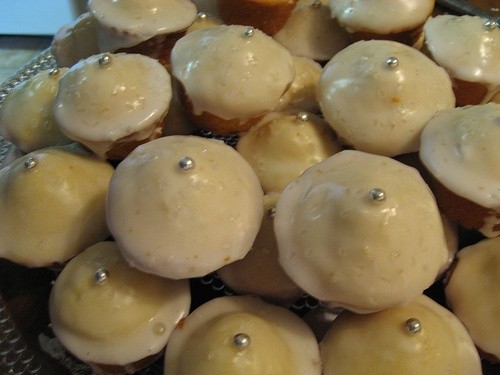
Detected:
[0,0,499,375]
